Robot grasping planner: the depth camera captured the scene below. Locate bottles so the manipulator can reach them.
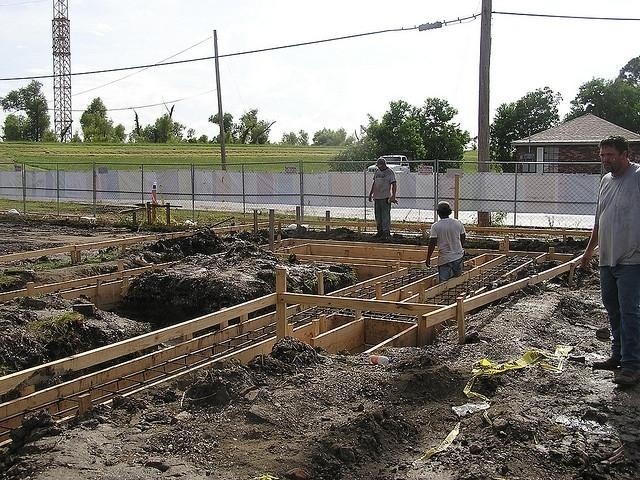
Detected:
[369,355,390,365]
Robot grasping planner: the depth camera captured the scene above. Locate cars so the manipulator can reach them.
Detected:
[366,155,410,173]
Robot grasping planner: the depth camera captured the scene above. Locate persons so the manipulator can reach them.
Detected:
[425,201,467,281]
[579,135,640,385]
[368,157,398,238]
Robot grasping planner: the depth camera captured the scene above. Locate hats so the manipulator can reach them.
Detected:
[375,157,387,168]
[437,201,452,212]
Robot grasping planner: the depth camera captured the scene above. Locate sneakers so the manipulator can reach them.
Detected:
[591,355,623,370]
[611,366,640,386]
